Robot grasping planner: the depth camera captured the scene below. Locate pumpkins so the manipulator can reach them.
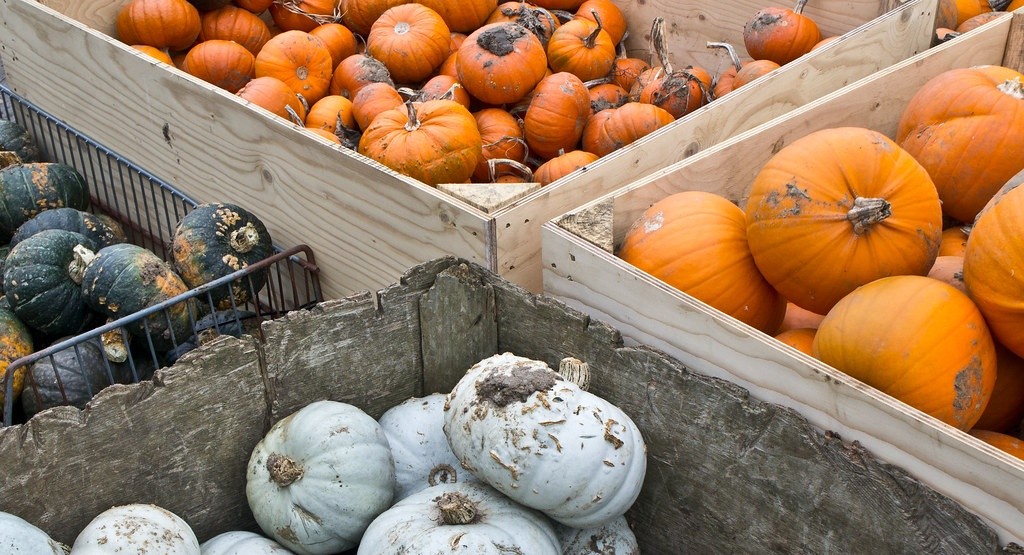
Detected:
[0,0,1024,555]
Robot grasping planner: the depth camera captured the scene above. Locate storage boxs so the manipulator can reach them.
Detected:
[0,0,938,318]
[541,6,1024,548]
[0,256,1024,555]
